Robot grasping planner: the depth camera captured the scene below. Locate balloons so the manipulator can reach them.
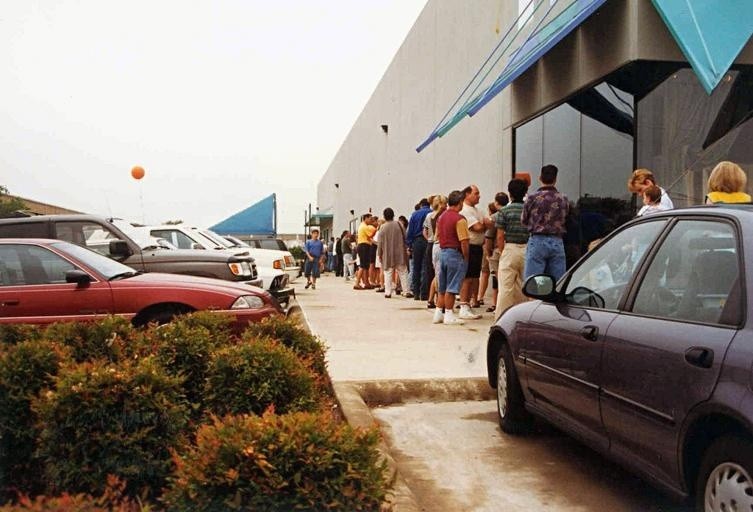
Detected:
[130,165,145,180]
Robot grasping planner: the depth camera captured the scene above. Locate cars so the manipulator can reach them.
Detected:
[483,201,753,511]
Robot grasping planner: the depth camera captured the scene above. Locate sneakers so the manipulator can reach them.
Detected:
[396,290,425,300]
[353,285,391,298]
[428,298,495,324]
[336,273,355,281]
[305,281,315,290]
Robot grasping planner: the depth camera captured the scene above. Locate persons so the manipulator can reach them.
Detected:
[476,164,568,325]
[403,185,485,325]
[627,169,673,211]
[704,160,751,205]
[348,208,414,299]
[301,229,357,289]
[638,185,661,215]
